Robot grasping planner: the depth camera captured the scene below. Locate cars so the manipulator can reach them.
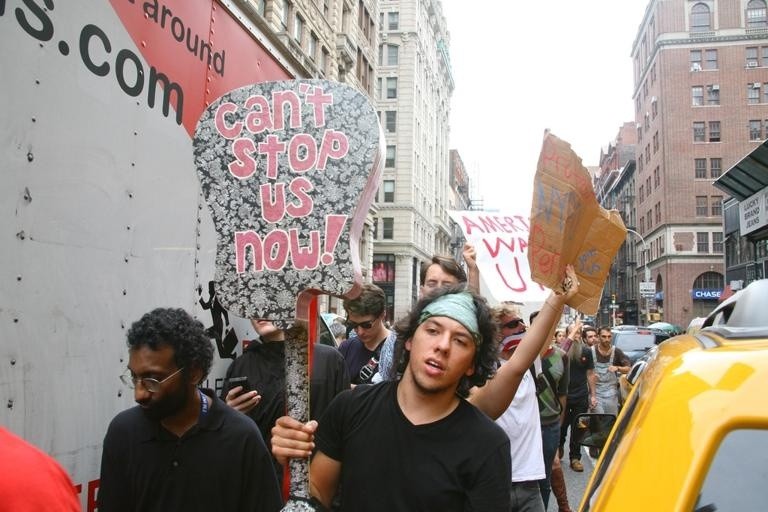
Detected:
[609,325,671,366]
[575,279,768,511]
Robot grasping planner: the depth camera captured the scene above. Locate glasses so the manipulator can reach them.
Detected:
[120,362,191,394]
[346,314,384,331]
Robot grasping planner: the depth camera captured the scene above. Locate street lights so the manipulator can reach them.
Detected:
[616,228,651,320]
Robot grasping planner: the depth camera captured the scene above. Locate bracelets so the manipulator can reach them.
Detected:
[544,301,562,316]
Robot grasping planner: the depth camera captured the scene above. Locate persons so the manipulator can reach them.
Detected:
[552,450,572,511]
[583,326,601,350]
[554,331,565,346]
[379,264,581,422]
[338,287,391,389]
[586,324,631,455]
[486,304,565,511]
[271,290,512,512]
[525,312,570,508]
[1,428,84,512]
[379,255,468,382]
[559,324,598,472]
[218,318,353,475]
[96,310,283,512]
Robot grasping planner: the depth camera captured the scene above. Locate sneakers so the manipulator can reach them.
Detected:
[573,460,584,473]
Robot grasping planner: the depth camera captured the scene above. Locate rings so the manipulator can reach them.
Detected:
[577,280,581,288]
